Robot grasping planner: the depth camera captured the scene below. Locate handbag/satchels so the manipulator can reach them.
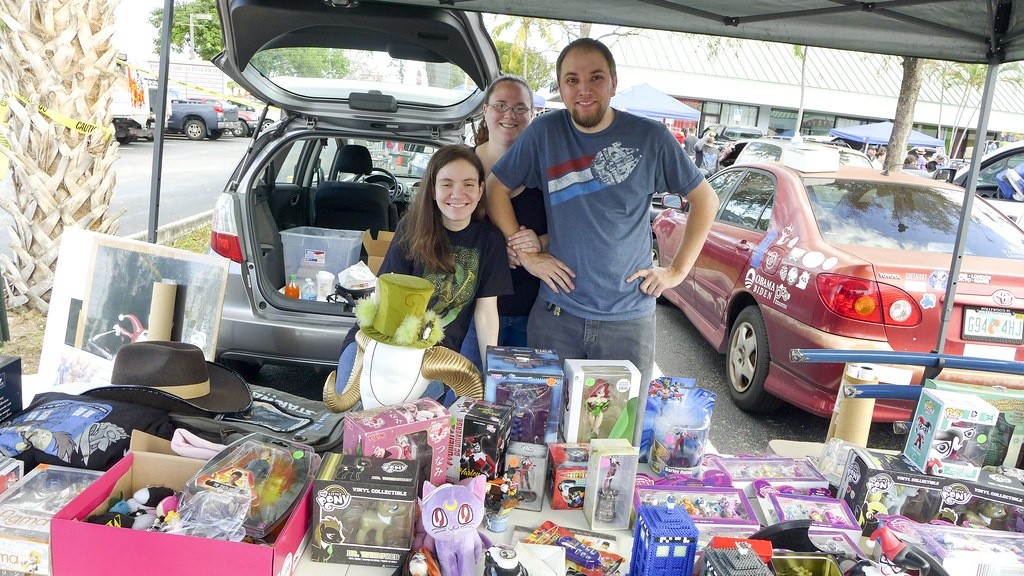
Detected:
[167,381,363,457]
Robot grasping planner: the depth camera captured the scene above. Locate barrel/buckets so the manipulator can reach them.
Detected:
[338,283,375,313]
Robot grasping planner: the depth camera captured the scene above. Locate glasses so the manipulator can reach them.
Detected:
[487,102,534,114]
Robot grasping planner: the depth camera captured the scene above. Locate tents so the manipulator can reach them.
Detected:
[827,118,946,156]
[606,79,701,138]
[148,0,1024,366]
[531,93,547,115]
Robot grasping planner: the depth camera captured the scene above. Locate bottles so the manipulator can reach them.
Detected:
[301,278,317,302]
[284,274,299,300]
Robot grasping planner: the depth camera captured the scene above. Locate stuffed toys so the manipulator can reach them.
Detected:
[418,474,487,576]
[323,274,483,414]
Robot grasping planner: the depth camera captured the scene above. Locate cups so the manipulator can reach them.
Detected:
[316,271,335,301]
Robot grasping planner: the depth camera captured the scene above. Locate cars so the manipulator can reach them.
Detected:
[112,80,283,146]
[197,1,506,386]
[926,140,1024,212]
[697,124,878,177]
[649,159,1024,423]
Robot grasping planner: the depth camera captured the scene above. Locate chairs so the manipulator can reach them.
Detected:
[314,146,390,241]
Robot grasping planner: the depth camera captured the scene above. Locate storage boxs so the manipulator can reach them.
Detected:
[280,226,365,286]
[360,229,395,275]
[0,346,1024,575]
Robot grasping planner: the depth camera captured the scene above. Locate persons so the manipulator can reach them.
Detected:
[674,126,718,175]
[584,379,611,443]
[242,450,272,482]
[866,145,948,179]
[519,457,536,492]
[333,145,515,410]
[604,455,620,489]
[446,75,557,411]
[486,38,721,473]
[224,472,241,488]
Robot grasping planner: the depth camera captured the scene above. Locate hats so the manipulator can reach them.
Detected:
[937,154,944,159]
[869,145,877,149]
[354,274,446,348]
[79,341,253,413]
